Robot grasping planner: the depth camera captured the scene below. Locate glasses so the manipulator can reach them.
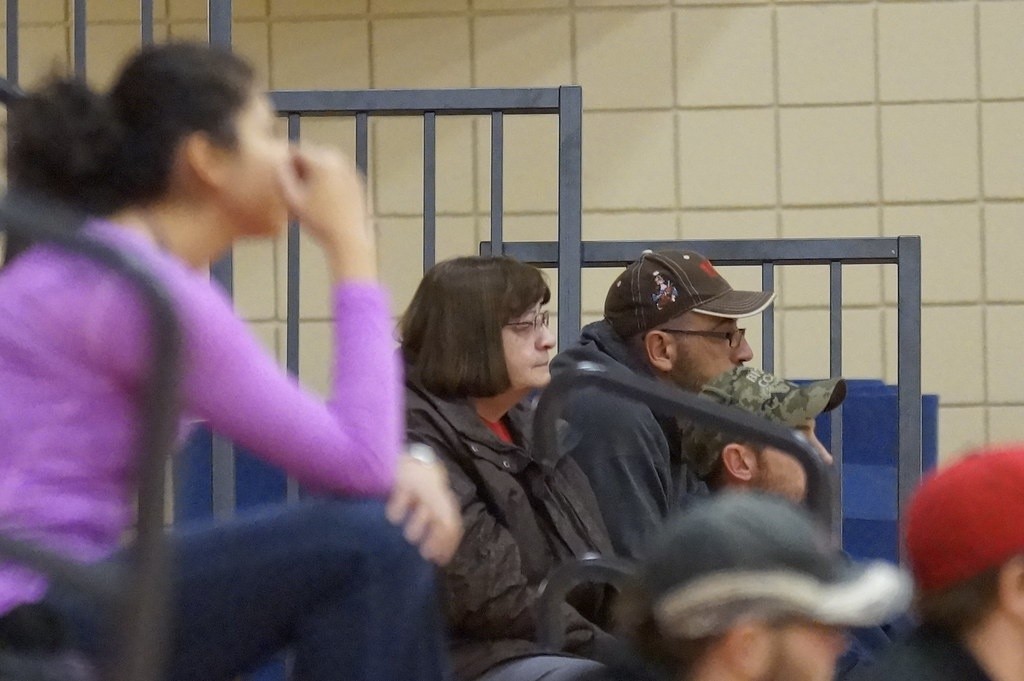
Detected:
[504,310,550,331]
[641,323,747,351]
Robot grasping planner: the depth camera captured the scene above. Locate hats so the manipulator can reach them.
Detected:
[639,491,911,638]
[605,249,774,343]
[900,444,1024,592]
[676,365,844,480]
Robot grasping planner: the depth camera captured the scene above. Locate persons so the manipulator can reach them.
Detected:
[533,248,777,560]
[0,41,464,679]
[400,257,620,681]
[681,367,892,681]
[610,490,898,681]
[843,446,1024,681]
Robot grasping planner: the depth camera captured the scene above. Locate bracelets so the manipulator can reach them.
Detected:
[411,443,436,463]
